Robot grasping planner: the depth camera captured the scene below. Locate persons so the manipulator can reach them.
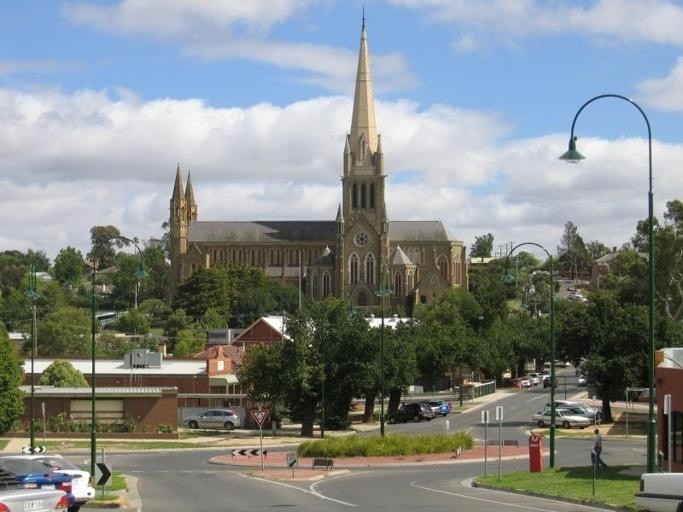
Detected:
[592,429,607,470]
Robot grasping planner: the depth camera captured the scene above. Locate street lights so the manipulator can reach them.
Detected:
[499,242,555,441]
[89,236,151,480]
[556,92,657,437]
[22,262,39,446]
[373,286,394,436]
[318,289,358,438]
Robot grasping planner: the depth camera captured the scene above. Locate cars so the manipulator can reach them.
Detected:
[532,398,603,429]
[0,452,96,512]
[182,409,242,430]
[509,372,558,388]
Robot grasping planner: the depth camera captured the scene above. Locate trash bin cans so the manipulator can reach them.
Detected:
[529,434,544,473]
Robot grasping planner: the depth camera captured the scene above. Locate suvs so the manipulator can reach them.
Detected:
[387,400,450,424]
